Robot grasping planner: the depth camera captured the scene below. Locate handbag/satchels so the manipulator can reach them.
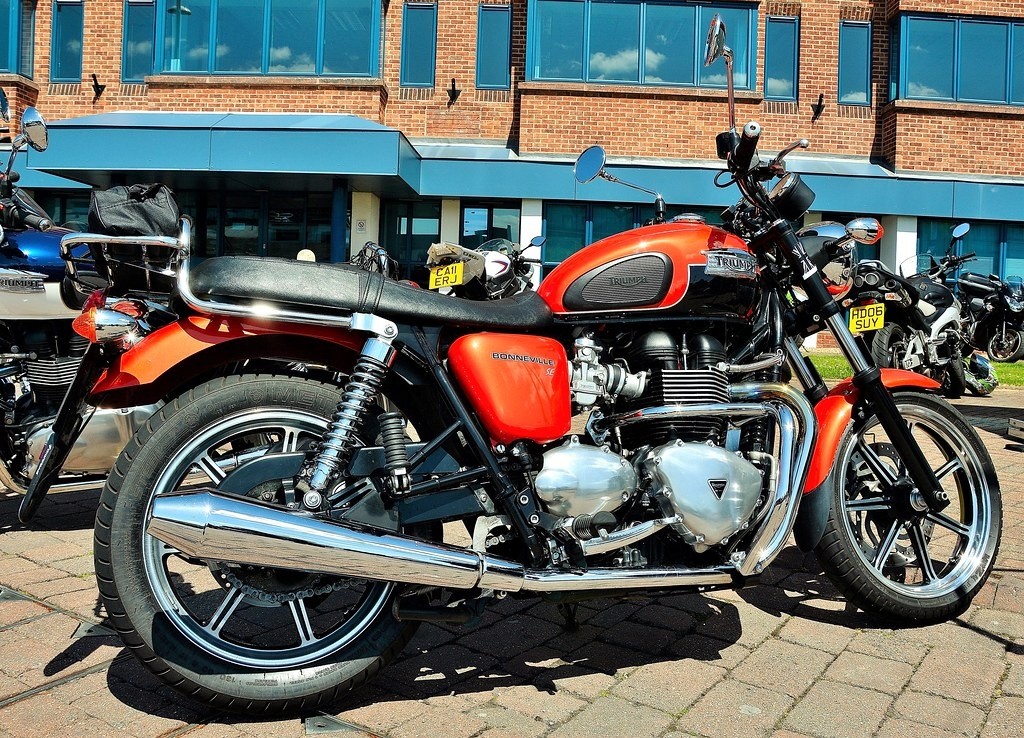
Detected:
[88,183,179,293]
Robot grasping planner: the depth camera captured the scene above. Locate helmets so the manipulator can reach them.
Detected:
[964,353,999,396]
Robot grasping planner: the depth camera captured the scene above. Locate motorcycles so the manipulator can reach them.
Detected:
[954,271,1024,363]
[57,11,1003,712]
[0,87,279,494]
[841,222,979,399]
[425,233,548,303]
